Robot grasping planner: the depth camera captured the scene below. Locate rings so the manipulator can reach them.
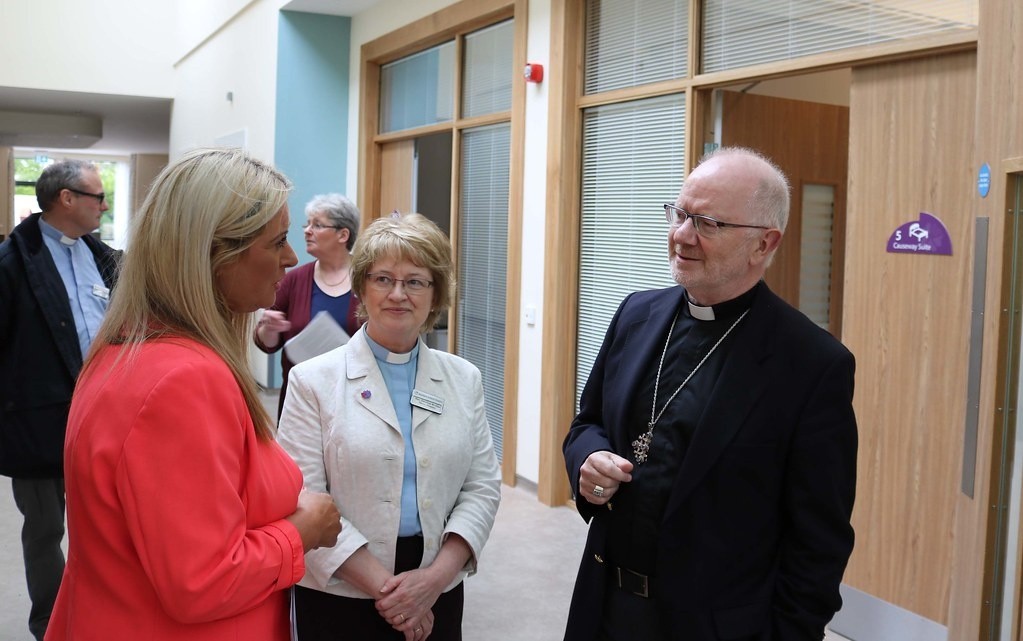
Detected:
[592,485,603,497]
[400,614,405,622]
[415,627,422,632]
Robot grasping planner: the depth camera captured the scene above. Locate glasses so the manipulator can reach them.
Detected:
[59,189,104,205]
[302,222,343,233]
[365,272,435,295]
[664,204,771,239]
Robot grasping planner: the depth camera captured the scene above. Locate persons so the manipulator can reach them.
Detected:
[251,192,370,430]
[0,160,124,641]
[562,146,858,641]
[44,147,344,641]
[276,212,501,641]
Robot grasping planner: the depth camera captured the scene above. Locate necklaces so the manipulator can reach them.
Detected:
[317,261,351,286]
[631,301,750,466]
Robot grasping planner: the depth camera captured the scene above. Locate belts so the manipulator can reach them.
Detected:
[607,563,682,598]
